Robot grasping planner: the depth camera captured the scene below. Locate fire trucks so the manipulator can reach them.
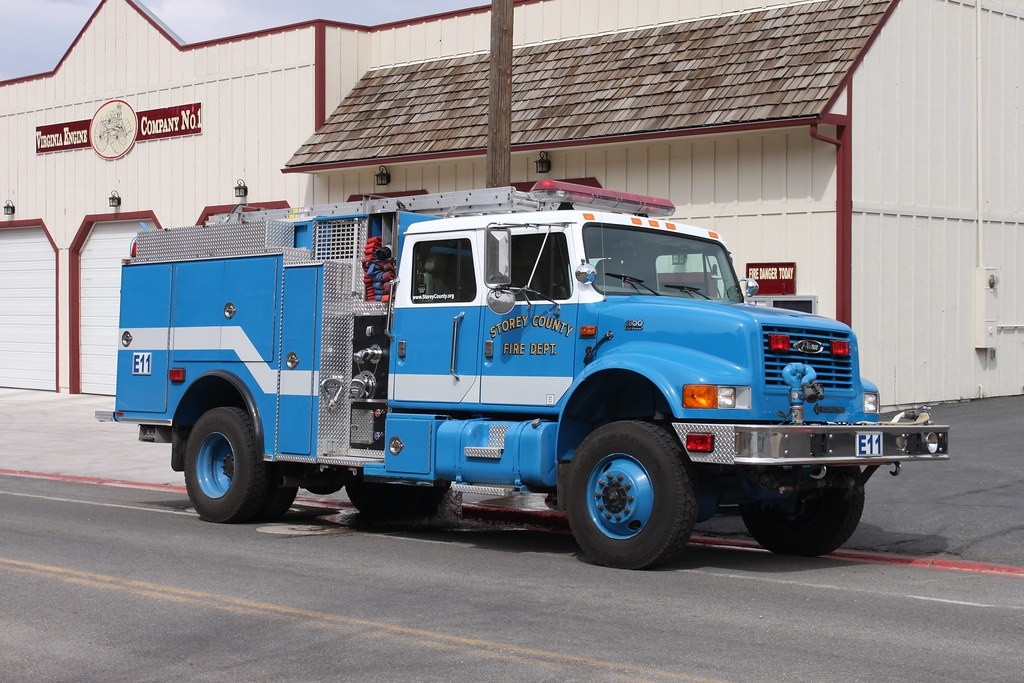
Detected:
[94,179,952,570]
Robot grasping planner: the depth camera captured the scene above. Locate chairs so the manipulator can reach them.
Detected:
[533,262,568,300]
[422,252,476,304]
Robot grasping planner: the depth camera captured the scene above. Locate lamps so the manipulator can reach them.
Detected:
[235,180,247,197]
[534,151,551,173]
[109,190,121,206]
[374,165,390,185]
[4,200,15,215]
